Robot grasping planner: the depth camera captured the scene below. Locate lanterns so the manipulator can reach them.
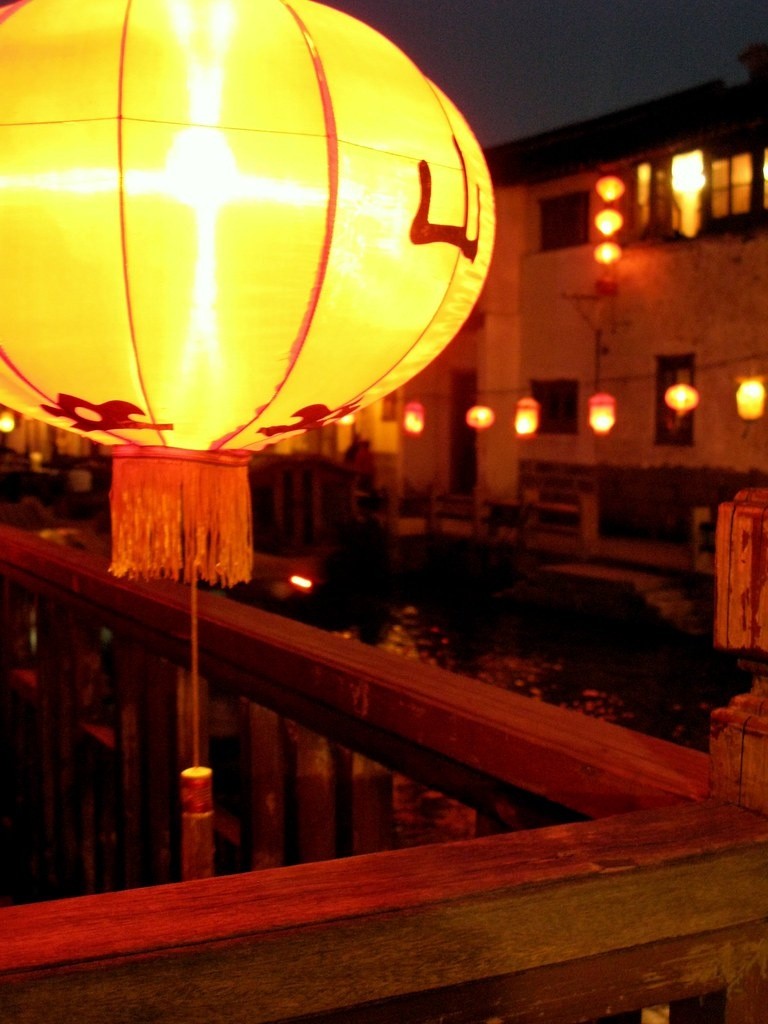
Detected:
[403,381,765,437]
[0,0,495,582]
[594,176,625,264]
